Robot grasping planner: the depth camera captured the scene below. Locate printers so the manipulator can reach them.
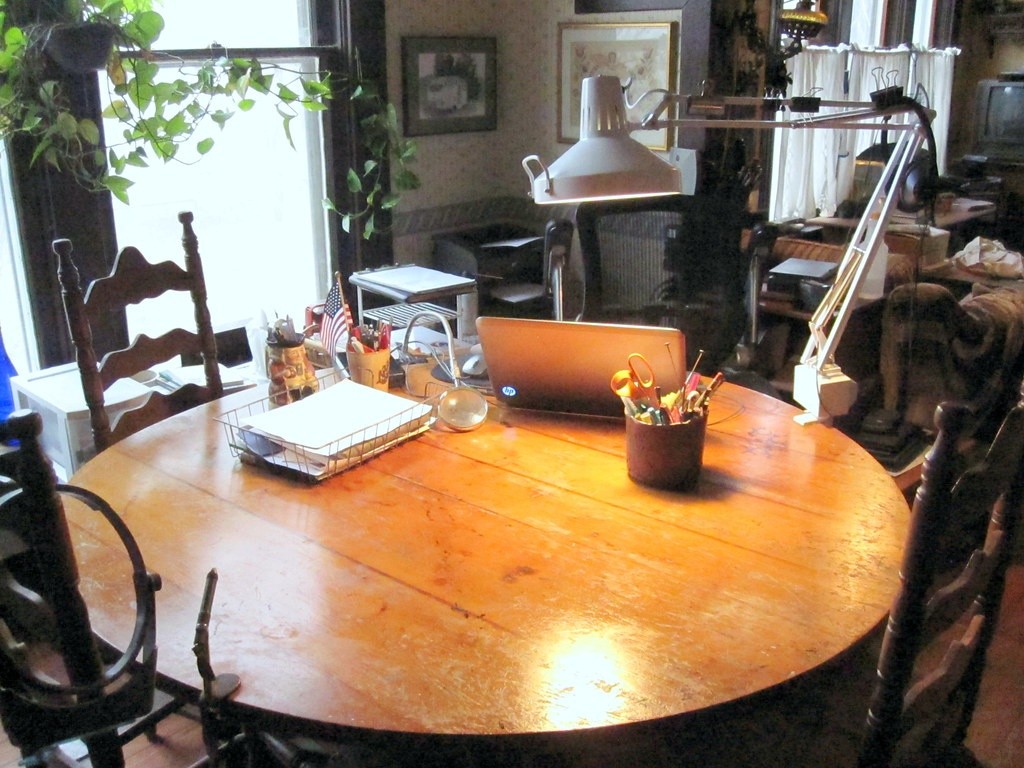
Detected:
[431,222,544,308]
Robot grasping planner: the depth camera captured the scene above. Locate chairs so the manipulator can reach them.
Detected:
[572,195,747,377]
[50,212,221,455]
[1,408,326,768]
[715,397,1024,766]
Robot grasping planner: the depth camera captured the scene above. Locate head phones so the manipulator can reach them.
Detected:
[881,95,938,214]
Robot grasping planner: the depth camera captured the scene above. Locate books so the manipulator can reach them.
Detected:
[348,264,478,304]
[236,375,432,466]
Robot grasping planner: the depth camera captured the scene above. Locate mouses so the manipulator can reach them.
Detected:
[462,355,489,377]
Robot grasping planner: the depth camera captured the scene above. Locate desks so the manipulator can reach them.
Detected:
[918,259,1024,299]
[755,293,885,379]
[56,366,916,768]
[808,197,997,255]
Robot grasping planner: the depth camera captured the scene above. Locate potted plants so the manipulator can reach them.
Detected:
[0,0,421,239]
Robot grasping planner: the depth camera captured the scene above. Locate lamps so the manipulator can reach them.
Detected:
[521,70,940,474]
[741,0,828,62]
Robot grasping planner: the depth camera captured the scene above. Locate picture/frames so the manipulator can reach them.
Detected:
[555,22,680,151]
[400,32,499,135]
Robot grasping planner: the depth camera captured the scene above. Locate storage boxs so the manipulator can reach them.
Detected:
[884,223,950,269]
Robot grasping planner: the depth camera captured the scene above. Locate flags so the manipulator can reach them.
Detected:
[321,276,355,356]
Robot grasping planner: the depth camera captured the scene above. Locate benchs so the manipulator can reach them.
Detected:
[741,230,912,354]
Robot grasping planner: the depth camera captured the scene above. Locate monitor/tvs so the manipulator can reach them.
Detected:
[971,79,1024,143]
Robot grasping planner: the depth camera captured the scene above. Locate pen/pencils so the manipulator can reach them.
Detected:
[694,372,724,407]
[641,386,671,424]
[349,319,393,353]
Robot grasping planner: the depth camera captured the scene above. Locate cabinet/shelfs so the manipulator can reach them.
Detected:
[961,155,1024,236]
[10,358,154,484]
[348,264,477,342]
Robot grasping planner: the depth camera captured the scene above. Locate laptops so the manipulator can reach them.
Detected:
[474,316,701,420]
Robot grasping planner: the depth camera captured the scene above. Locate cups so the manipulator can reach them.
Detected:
[346,345,390,393]
[624,408,708,486]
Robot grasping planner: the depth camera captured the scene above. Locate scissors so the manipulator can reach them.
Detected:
[611,353,659,407]
